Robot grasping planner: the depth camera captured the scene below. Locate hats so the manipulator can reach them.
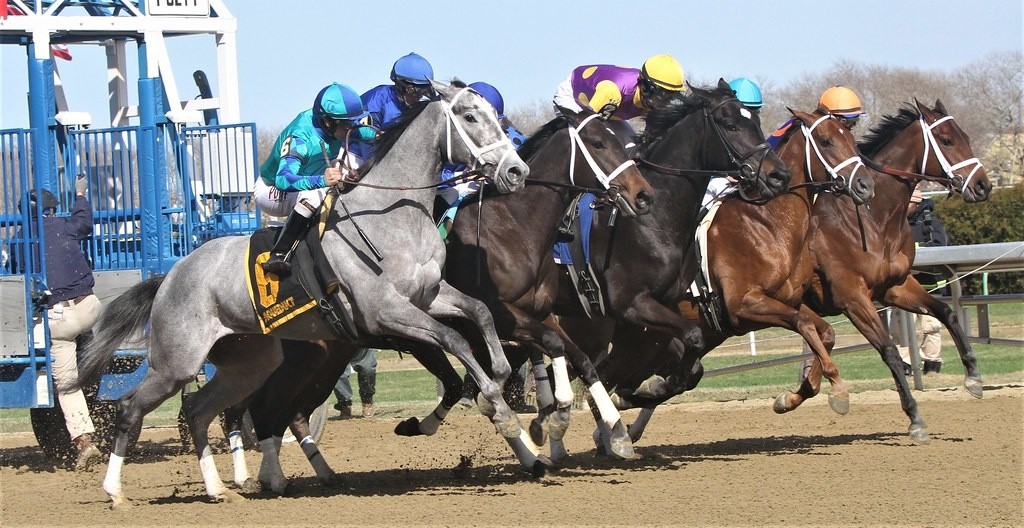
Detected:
[910,183,922,203]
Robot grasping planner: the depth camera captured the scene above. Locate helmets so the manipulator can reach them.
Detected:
[468,82,507,118]
[313,82,369,121]
[728,78,765,107]
[641,54,686,92]
[819,85,865,115]
[18,188,60,217]
[390,52,434,87]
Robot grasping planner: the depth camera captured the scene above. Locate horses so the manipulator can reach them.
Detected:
[225,100,655,482]
[626,97,993,446]
[57,74,558,512]
[393,77,791,457]
[593,103,875,415]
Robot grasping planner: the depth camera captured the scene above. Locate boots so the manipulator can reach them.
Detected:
[262,207,310,277]
[924,361,941,374]
[73,434,102,471]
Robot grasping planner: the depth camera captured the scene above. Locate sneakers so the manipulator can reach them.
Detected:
[363,402,375,418]
[340,405,352,418]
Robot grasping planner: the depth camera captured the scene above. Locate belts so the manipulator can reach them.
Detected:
[63,295,85,307]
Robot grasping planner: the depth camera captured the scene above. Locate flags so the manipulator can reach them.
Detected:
[0,0,72,61]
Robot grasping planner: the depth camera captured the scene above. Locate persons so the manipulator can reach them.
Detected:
[766,85,866,154]
[552,54,687,149]
[253,82,380,275]
[333,347,377,419]
[5,174,102,474]
[334,52,433,181]
[433,80,528,217]
[889,184,947,376]
[695,77,765,222]
